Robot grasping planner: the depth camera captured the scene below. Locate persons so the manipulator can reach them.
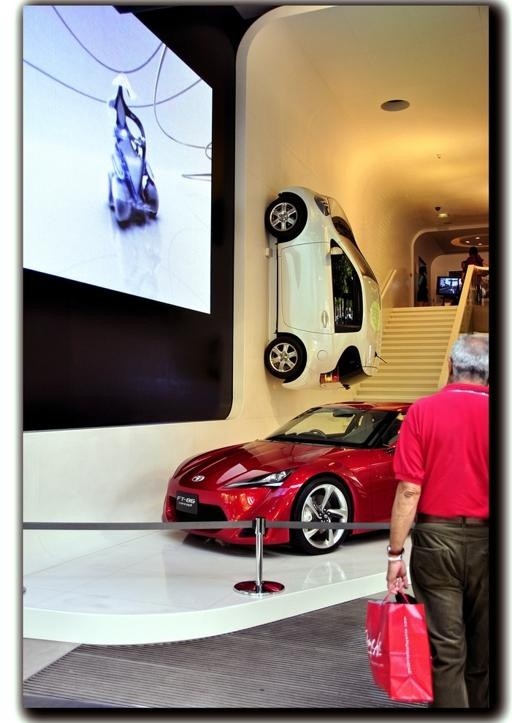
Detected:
[416,266,429,307]
[463,246,483,304]
[386,334,492,710]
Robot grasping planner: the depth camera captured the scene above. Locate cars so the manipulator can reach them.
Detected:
[265,186,388,389]
[164,400,414,553]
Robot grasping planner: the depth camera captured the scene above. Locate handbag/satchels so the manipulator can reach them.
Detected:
[365,586,434,705]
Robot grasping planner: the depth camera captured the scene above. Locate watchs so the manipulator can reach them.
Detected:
[387,545,405,555]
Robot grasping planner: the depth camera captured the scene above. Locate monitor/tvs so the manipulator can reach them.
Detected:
[436,276,462,296]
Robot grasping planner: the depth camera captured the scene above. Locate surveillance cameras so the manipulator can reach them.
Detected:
[435,207,441,211]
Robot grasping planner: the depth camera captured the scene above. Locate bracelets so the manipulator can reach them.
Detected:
[386,553,402,561]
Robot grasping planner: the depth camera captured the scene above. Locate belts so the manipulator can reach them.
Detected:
[416,514,489,528]
